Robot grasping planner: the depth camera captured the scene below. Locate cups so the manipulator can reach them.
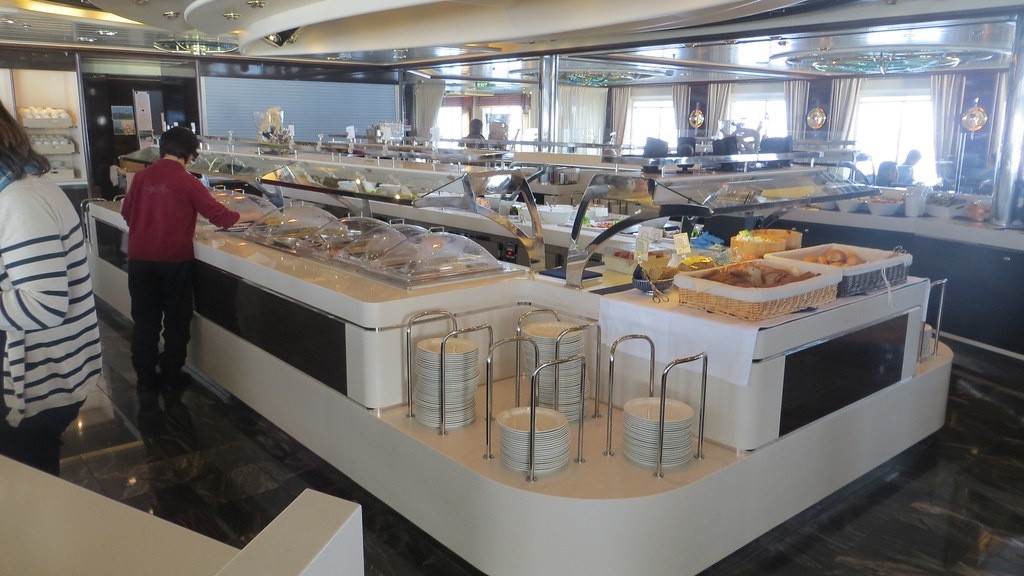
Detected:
[905,195,927,217]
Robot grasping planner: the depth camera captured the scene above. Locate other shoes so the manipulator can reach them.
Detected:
[139,372,161,389]
[162,369,177,398]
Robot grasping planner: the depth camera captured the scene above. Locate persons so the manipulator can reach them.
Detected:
[0,101,105,477]
[120,127,266,428]
[457,119,489,199]
[900,149,921,185]
[876,161,898,188]
[933,155,998,197]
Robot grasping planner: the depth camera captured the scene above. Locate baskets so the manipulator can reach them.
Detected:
[838,264,908,298]
[674,259,838,322]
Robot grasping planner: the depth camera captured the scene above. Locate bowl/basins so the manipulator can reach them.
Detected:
[485,193,574,226]
[338,179,399,199]
[642,217,670,229]
[808,198,903,216]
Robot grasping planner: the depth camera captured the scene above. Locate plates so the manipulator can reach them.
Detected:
[412,337,480,429]
[619,225,704,244]
[622,396,694,467]
[494,322,590,472]
[582,221,617,231]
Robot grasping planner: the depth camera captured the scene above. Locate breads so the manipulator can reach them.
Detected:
[650,229,867,288]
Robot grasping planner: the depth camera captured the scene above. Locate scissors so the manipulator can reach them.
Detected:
[642,270,670,303]
[885,245,907,259]
